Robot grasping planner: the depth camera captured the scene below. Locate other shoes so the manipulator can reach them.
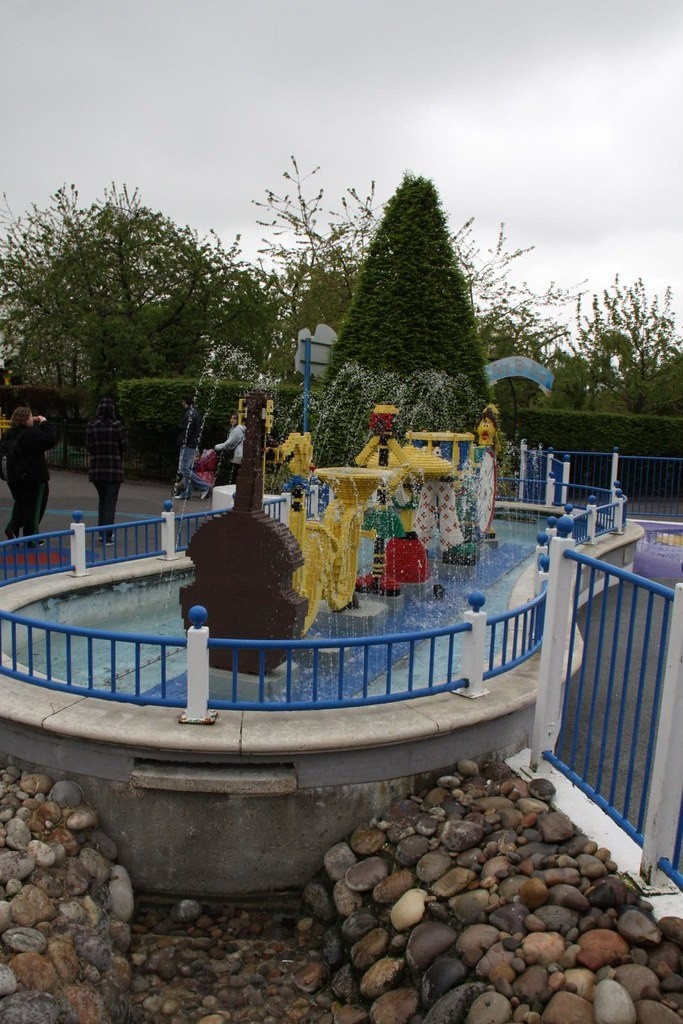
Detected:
[173,493,190,499]
[201,484,212,499]
[97,534,117,546]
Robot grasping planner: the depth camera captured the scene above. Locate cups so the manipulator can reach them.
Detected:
[33,417,40,422]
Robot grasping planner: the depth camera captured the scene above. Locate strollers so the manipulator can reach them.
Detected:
[170,447,219,498]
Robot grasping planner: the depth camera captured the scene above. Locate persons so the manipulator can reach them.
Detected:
[187,450,201,470]
[213,413,246,484]
[87,397,128,545]
[0,407,57,547]
[174,396,212,499]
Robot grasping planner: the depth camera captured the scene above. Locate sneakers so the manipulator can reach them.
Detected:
[25,539,44,547]
[4,526,19,547]
